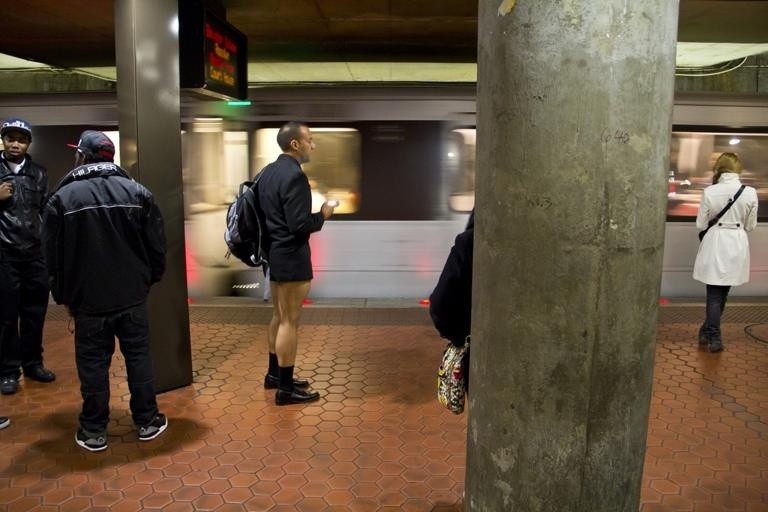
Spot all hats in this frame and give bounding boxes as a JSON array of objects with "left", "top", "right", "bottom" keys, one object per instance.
[
  {"left": 67, "top": 130, "right": 115, "bottom": 161},
  {"left": 0, "top": 117, "right": 33, "bottom": 140}
]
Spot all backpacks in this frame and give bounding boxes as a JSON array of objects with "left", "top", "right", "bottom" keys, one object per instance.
[{"left": 224, "top": 163, "right": 270, "bottom": 268}]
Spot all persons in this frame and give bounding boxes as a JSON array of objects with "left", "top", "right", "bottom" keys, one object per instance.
[
  {"left": 429, "top": 207, "right": 474, "bottom": 397},
  {"left": 253, "top": 120, "right": 340, "bottom": 405},
  {"left": 41, "top": 130, "right": 169, "bottom": 452},
  {"left": 692, "top": 152, "right": 758, "bottom": 352},
  {"left": 0, "top": 117, "right": 56, "bottom": 394}
]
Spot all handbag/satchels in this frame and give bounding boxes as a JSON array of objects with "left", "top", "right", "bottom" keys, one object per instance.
[{"left": 437, "top": 335, "right": 473, "bottom": 416}]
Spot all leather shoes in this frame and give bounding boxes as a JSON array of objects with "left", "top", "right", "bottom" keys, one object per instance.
[
  {"left": 275, "top": 387, "right": 319, "bottom": 405},
  {"left": 0, "top": 375, "right": 18, "bottom": 394},
  {"left": 25, "top": 368, "right": 56, "bottom": 382},
  {"left": 265, "top": 373, "right": 309, "bottom": 388}
]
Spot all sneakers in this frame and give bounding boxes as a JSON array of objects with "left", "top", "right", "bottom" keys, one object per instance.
[
  {"left": 698, "top": 334, "right": 722, "bottom": 353},
  {"left": 74, "top": 430, "right": 108, "bottom": 451},
  {"left": 138, "top": 413, "right": 168, "bottom": 441}
]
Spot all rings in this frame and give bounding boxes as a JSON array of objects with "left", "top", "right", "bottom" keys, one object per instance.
[{"left": 8, "top": 189, "right": 10, "bottom": 193}]
